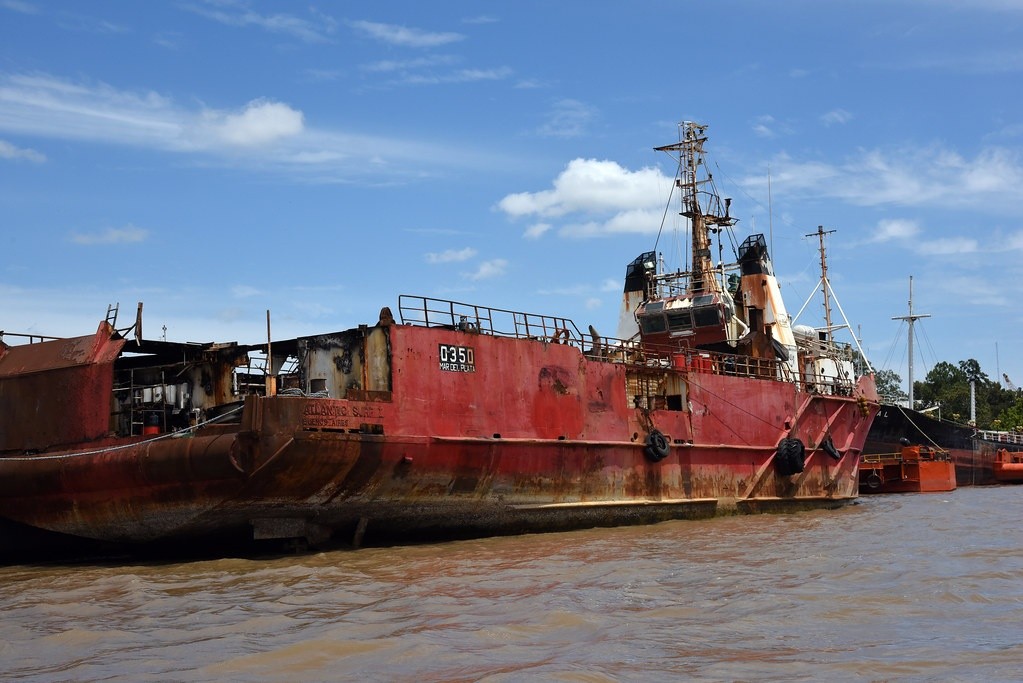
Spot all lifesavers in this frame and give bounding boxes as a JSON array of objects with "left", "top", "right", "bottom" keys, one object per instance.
[
  {"left": 824, "top": 441, "right": 840, "bottom": 460},
  {"left": 866, "top": 475, "right": 882, "bottom": 489},
  {"left": 553, "top": 329, "right": 570, "bottom": 345},
  {"left": 777, "top": 438, "right": 806, "bottom": 476},
  {"left": 645, "top": 432, "right": 670, "bottom": 463}
]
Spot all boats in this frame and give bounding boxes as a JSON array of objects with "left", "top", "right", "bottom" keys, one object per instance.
[
  {"left": 859, "top": 275, "right": 1023, "bottom": 493},
  {"left": 991, "top": 447, "right": 1023, "bottom": 483},
  {"left": 0, "top": 121, "right": 880, "bottom": 557}
]
